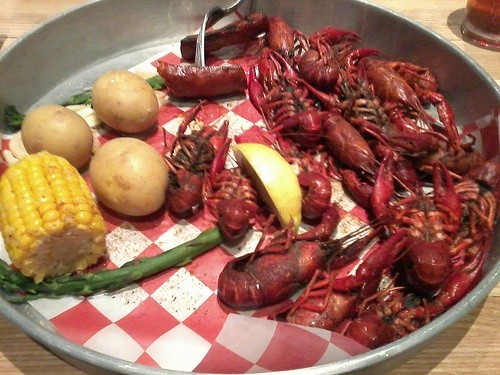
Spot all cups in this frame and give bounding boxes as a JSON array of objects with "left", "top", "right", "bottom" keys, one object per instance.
[{"left": 460, "top": 0, "right": 500, "bottom": 51}]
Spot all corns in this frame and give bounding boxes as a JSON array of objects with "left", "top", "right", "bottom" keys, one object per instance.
[{"left": 1, "top": 151, "right": 109, "bottom": 283}]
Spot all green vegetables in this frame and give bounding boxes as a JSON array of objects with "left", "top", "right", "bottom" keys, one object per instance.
[
  {"left": 0, "top": 227, "right": 225, "bottom": 304},
  {"left": 4, "top": 73, "right": 167, "bottom": 134}
]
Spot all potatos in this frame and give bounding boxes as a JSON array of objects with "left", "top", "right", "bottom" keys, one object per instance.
[
  {"left": 21, "top": 69, "right": 169, "bottom": 217},
  {"left": 231, "top": 143, "right": 303, "bottom": 235}
]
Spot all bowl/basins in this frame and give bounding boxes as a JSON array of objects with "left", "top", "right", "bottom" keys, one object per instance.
[{"left": 1, "top": 0, "right": 500, "bottom": 375}]
